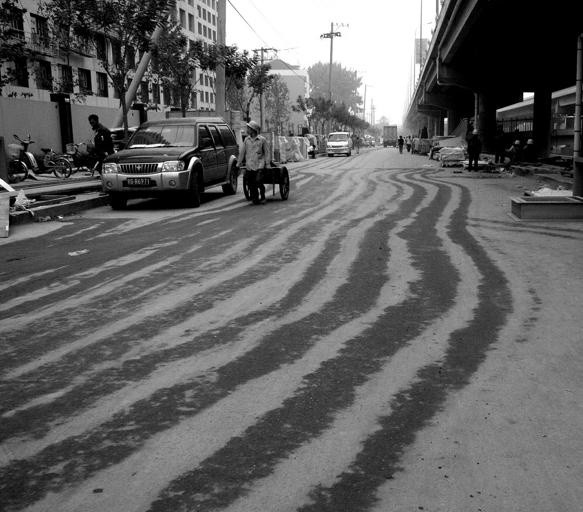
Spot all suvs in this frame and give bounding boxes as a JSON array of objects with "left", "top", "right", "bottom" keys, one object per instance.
[{"left": 101, "top": 115, "right": 241, "bottom": 210}]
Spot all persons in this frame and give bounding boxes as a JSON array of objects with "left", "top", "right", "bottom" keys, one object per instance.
[
  {"left": 398, "top": 135, "right": 420, "bottom": 154},
  {"left": 236, "top": 121, "right": 272, "bottom": 206},
  {"left": 493, "top": 125, "right": 539, "bottom": 163},
  {"left": 465, "top": 128, "right": 482, "bottom": 172},
  {"left": 88, "top": 114, "right": 115, "bottom": 175},
  {"left": 354, "top": 137, "right": 360, "bottom": 154}
]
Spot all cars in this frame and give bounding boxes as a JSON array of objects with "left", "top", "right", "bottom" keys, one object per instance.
[
  {"left": 365, "top": 136, "right": 375, "bottom": 148},
  {"left": 74, "top": 127, "right": 136, "bottom": 176}
]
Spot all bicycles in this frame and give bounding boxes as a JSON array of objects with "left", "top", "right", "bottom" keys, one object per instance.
[{"left": 59, "top": 143, "right": 101, "bottom": 175}]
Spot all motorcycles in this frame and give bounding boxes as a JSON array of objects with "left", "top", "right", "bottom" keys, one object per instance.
[{"left": 8, "top": 133, "right": 71, "bottom": 183}]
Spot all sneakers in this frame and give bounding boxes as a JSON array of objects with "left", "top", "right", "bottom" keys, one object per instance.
[{"left": 249, "top": 199, "right": 266, "bottom": 205}]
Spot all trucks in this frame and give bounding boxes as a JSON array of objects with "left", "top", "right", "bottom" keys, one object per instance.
[{"left": 383, "top": 126, "right": 396, "bottom": 148}]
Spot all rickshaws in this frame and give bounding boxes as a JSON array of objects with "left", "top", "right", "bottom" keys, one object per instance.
[{"left": 238, "top": 160, "right": 290, "bottom": 203}]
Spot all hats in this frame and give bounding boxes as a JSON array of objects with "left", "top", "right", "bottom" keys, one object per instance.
[{"left": 246, "top": 120, "right": 260, "bottom": 131}]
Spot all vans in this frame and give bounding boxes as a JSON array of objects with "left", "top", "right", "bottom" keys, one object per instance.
[{"left": 326, "top": 132, "right": 352, "bottom": 157}]
